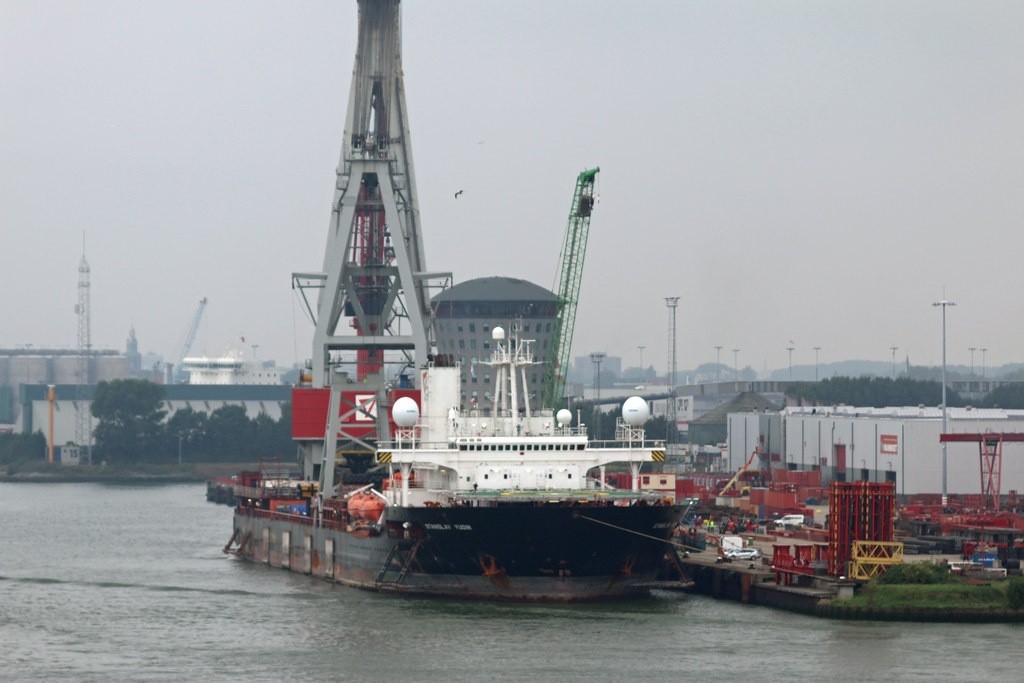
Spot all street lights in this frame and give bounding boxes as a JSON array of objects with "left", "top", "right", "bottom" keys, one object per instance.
[
  {"left": 179, "top": 437, "right": 184, "bottom": 464},
  {"left": 638, "top": 346, "right": 646, "bottom": 385},
  {"left": 932, "top": 284, "right": 956, "bottom": 505},
  {"left": 890, "top": 346, "right": 899, "bottom": 379},
  {"left": 25, "top": 344, "right": 32, "bottom": 384},
  {"left": 714, "top": 346, "right": 722, "bottom": 383},
  {"left": 732, "top": 349, "right": 740, "bottom": 381},
  {"left": 787, "top": 348, "right": 795, "bottom": 381},
  {"left": 813, "top": 347, "right": 822, "bottom": 381},
  {"left": 968, "top": 347, "right": 976, "bottom": 376},
  {"left": 980, "top": 349, "right": 988, "bottom": 377}
]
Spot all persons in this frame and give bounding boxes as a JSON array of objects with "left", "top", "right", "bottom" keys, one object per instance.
[
  {"left": 707, "top": 519, "right": 715, "bottom": 533},
  {"left": 393, "top": 469, "right": 414, "bottom": 488},
  {"left": 607, "top": 476, "right": 617, "bottom": 487}
]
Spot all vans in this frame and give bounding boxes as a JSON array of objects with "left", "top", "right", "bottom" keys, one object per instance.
[{"left": 774, "top": 514, "right": 804, "bottom": 527}]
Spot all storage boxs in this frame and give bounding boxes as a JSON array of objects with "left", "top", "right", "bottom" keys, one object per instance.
[{"left": 604, "top": 468, "right": 827, "bottom": 524}]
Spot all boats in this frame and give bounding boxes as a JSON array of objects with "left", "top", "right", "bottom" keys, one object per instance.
[{"left": 222, "top": 313, "right": 695, "bottom": 603}]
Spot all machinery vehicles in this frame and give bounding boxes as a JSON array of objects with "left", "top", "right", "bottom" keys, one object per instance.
[{"left": 712, "top": 446, "right": 763, "bottom": 497}]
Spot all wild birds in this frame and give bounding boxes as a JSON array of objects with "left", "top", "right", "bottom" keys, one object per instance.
[{"left": 454, "top": 190, "right": 463, "bottom": 199}]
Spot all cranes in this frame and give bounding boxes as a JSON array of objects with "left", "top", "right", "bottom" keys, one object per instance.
[
  {"left": 541, "top": 166, "right": 600, "bottom": 410},
  {"left": 173, "top": 297, "right": 207, "bottom": 384}
]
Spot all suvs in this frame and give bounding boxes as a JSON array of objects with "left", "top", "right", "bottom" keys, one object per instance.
[{"left": 724, "top": 548, "right": 760, "bottom": 561}]
